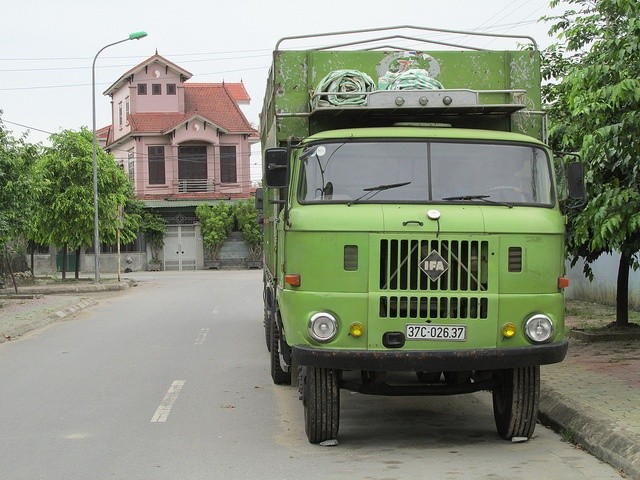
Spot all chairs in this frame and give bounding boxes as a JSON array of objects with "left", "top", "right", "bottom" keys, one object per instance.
[
  {"left": 448, "top": 175, "right": 486, "bottom": 202},
  {"left": 346, "top": 174, "right": 384, "bottom": 200}
]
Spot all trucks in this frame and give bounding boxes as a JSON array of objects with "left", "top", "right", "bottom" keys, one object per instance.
[{"left": 257, "top": 24, "right": 590, "bottom": 446}]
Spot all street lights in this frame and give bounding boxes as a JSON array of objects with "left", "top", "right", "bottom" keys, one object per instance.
[{"left": 83, "top": 30, "right": 150, "bottom": 286}]
